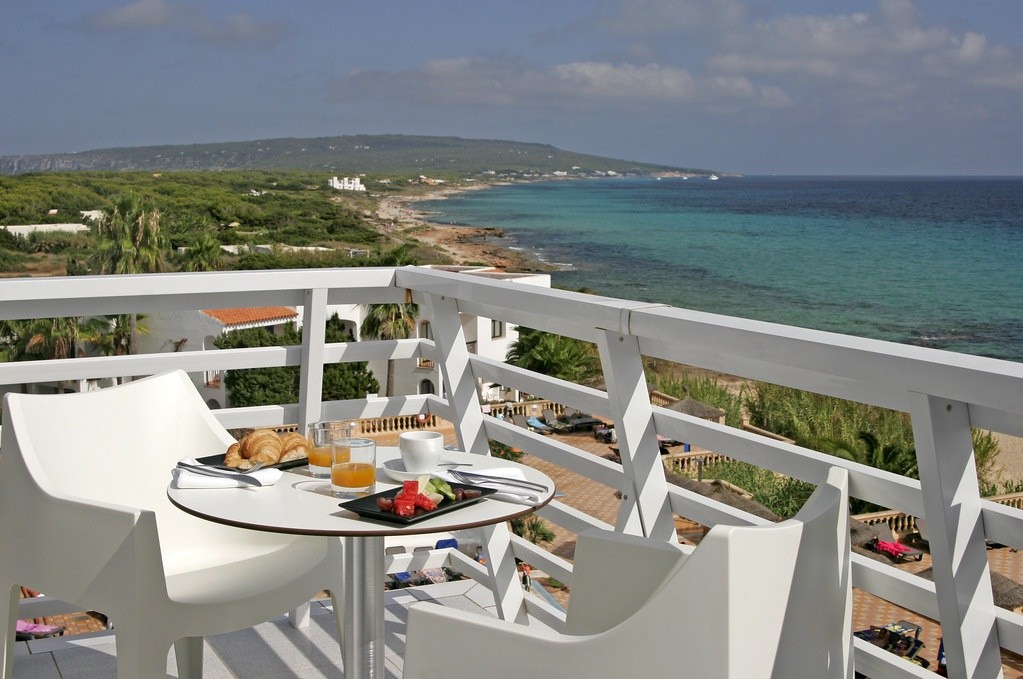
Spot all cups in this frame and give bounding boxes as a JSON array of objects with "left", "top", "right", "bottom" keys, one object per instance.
[
  {"left": 399, "top": 431, "right": 444, "bottom": 472},
  {"left": 330, "top": 437, "right": 376, "bottom": 500},
  {"left": 307, "top": 421, "right": 352, "bottom": 478}
]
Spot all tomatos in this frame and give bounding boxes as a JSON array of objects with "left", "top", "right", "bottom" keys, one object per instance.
[{"left": 393, "top": 480, "right": 436, "bottom": 517}]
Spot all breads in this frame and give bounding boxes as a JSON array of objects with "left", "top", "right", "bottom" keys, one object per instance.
[{"left": 222, "top": 427, "right": 310, "bottom": 471}]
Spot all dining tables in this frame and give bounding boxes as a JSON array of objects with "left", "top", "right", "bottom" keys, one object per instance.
[{"left": 165, "top": 444, "right": 557, "bottom": 679}]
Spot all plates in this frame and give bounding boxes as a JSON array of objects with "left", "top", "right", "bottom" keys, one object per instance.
[
  {"left": 338, "top": 481, "right": 499, "bottom": 525},
  {"left": 195, "top": 453, "right": 308, "bottom": 474},
  {"left": 382, "top": 458, "right": 458, "bottom": 482}
]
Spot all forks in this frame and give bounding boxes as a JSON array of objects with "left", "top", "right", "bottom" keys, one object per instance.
[
  {"left": 447, "top": 469, "right": 543, "bottom": 494},
  {"left": 195, "top": 461, "right": 265, "bottom": 474}
]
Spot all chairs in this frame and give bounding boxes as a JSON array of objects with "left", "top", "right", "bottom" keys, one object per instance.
[
  {"left": 866, "top": 522, "right": 924, "bottom": 562},
  {"left": 402, "top": 516, "right": 806, "bottom": 678},
  {"left": 914, "top": 518, "right": 929, "bottom": 546},
  {"left": 497, "top": 407, "right": 685, "bottom": 461},
  {"left": 562, "top": 464, "right": 857, "bottom": 679},
  {"left": 852, "top": 620, "right": 947, "bottom": 676},
  {"left": 0, "top": 368, "right": 354, "bottom": 679}
]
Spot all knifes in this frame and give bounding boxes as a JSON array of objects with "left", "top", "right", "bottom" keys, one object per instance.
[
  {"left": 177, "top": 461, "right": 262, "bottom": 487},
  {"left": 448, "top": 470, "right": 548, "bottom": 492}
]
